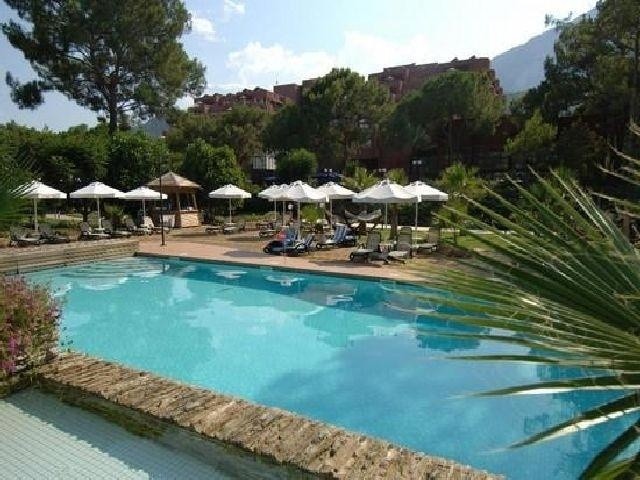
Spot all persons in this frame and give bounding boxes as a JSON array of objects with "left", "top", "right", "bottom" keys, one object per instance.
[{"left": 263, "top": 230, "right": 286, "bottom": 253}]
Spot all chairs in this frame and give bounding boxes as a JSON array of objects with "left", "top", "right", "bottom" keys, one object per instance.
[
  {"left": 9, "top": 216, "right": 170, "bottom": 248},
  {"left": 205, "top": 215, "right": 441, "bottom": 268}
]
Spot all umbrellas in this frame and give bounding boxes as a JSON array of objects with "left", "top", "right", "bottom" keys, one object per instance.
[
  {"left": 46, "top": 258, "right": 171, "bottom": 298},
  {"left": 7, "top": 178, "right": 68, "bottom": 235},
  {"left": 257, "top": 178, "right": 449, "bottom": 245},
  {"left": 214, "top": 267, "right": 248, "bottom": 280},
  {"left": 120, "top": 185, "right": 169, "bottom": 218},
  {"left": 384, "top": 295, "right": 435, "bottom": 317},
  {"left": 69, "top": 181, "right": 124, "bottom": 228},
  {"left": 208, "top": 183, "right": 252, "bottom": 223},
  {"left": 261, "top": 269, "right": 310, "bottom": 286}
]
